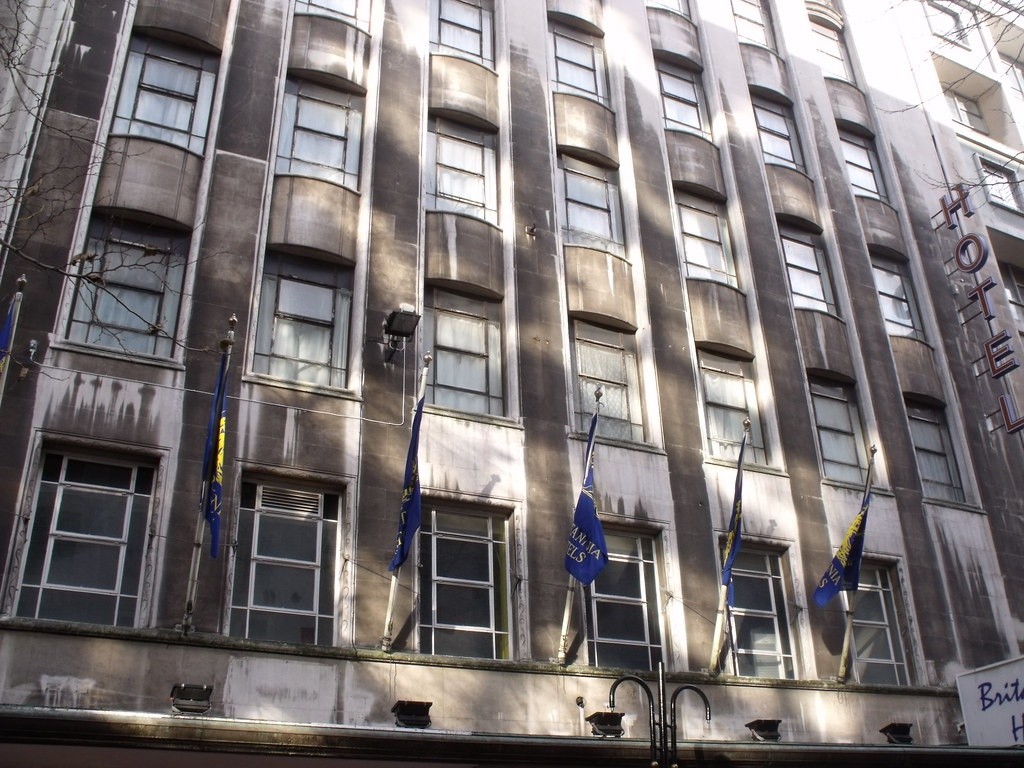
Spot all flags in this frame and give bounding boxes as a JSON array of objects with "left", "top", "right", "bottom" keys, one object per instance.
[
  {"left": 387, "top": 392, "right": 426, "bottom": 572},
  {"left": 721, "top": 434, "right": 745, "bottom": 607},
  {"left": 564, "top": 414, "right": 609, "bottom": 586},
  {"left": 201, "top": 350, "right": 228, "bottom": 561},
  {"left": 812, "top": 465, "right": 872, "bottom": 609}
]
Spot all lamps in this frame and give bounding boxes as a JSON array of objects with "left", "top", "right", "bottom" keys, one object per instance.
[
  {"left": 879, "top": 722, "right": 913, "bottom": 745},
  {"left": 390, "top": 700, "right": 433, "bottom": 728},
  {"left": 169, "top": 683, "right": 214, "bottom": 719},
  {"left": 745, "top": 719, "right": 782, "bottom": 742},
  {"left": 584, "top": 712, "right": 625, "bottom": 737},
  {"left": 381, "top": 303, "right": 422, "bottom": 363}
]
